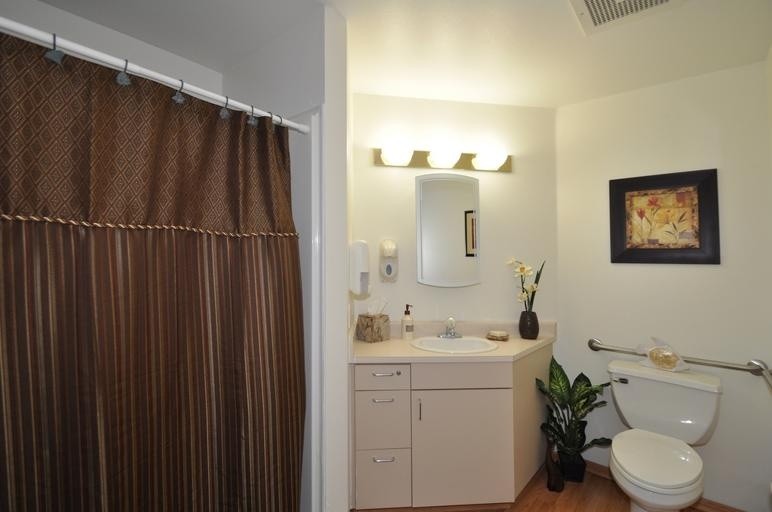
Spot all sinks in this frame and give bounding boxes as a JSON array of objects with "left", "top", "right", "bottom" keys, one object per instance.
[{"left": 410, "top": 336, "right": 497, "bottom": 354}]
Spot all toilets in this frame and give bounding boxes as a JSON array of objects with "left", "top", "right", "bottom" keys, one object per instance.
[{"left": 606, "top": 361, "right": 722, "bottom": 512}]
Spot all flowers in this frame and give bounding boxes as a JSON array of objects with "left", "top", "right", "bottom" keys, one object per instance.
[{"left": 506, "top": 257, "right": 546, "bottom": 312}]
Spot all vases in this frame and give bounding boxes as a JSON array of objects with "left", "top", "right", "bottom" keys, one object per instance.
[{"left": 519, "top": 310, "right": 539, "bottom": 340}]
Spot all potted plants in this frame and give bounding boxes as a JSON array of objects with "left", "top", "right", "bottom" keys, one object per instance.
[{"left": 536, "top": 355, "right": 613, "bottom": 483}]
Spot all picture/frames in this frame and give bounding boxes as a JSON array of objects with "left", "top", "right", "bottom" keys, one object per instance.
[
  {"left": 608, "top": 169, "right": 721, "bottom": 266},
  {"left": 464, "top": 210, "right": 475, "bottom": 256}
]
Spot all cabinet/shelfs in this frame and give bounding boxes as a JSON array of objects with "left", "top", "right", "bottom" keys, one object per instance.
[{"left": 349, "top": 344, "right": 553, "bottom": 509}]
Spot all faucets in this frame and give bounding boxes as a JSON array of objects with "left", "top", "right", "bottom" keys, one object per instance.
[{"left": 445, "top": 317, "right": 456, "bottom": 337}]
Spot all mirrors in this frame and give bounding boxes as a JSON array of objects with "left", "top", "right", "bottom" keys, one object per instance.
[{"left": 416, "top": 172, "right": 482, "bottom": 288}]
